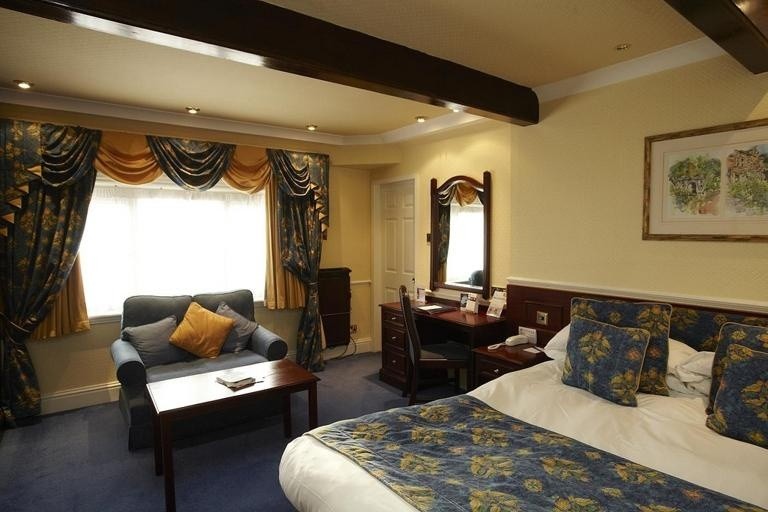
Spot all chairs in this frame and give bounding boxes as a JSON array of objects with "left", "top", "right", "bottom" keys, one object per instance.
[{"left": 397, "top": 282, "right": 472, "bottom": 409}]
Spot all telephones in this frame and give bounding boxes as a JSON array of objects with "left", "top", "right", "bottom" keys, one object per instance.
[{"left": 505, "top": 335, "right": 529, "bottom": 347}]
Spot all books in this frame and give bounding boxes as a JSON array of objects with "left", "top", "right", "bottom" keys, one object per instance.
[
  {"left": 413, "top": 302, "right": 458, "bottom": 318},
  {"left": 216, "top": 370, "right": 257, "bottom": 389}
]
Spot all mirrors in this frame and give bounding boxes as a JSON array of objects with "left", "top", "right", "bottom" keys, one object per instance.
[{"left": 427, "top": 170, "right": 494, "bottom": 300}]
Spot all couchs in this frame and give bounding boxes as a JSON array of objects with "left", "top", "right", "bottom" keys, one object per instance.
[{"left": 108, "top": 288, "right": 290, "bottom": 453}]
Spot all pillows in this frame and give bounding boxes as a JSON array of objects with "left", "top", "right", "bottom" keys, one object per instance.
[
  {"left": 122, "top": 315, "right": 188, "bottom": 369},
  {"left": 214, "top": 300, "right": 258, "bottom": 357},
  {"left": 541, "top": 294, "right": 768, "bottom": 451},
  {"left": 164, "top": 300, "right": 236, "bottom": 363}
]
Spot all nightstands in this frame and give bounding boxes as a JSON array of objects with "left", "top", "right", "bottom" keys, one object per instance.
[{"left": 470, "top": 334, "right": 554, "bottom": 389}]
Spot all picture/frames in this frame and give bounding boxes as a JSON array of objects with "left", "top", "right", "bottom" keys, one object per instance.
[{"left": 640, "top": 116, "right": 768, "bottom": 243}]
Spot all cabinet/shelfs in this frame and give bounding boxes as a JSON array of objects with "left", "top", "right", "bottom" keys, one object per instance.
[{"left": 316, "top": 267, "right": 352, "bottom": 349}]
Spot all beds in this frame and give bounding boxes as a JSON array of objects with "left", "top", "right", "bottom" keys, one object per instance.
[{"left": 278, "top": 294, "right": 768, "bottom": 512}]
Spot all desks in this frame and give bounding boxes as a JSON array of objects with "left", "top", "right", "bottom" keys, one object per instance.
[
  {"left": 145, "top": 356, "right": 322, "bottom": 511},
  {"left": 378, "top": 297, "right": 511, "bottom": 396}
]
